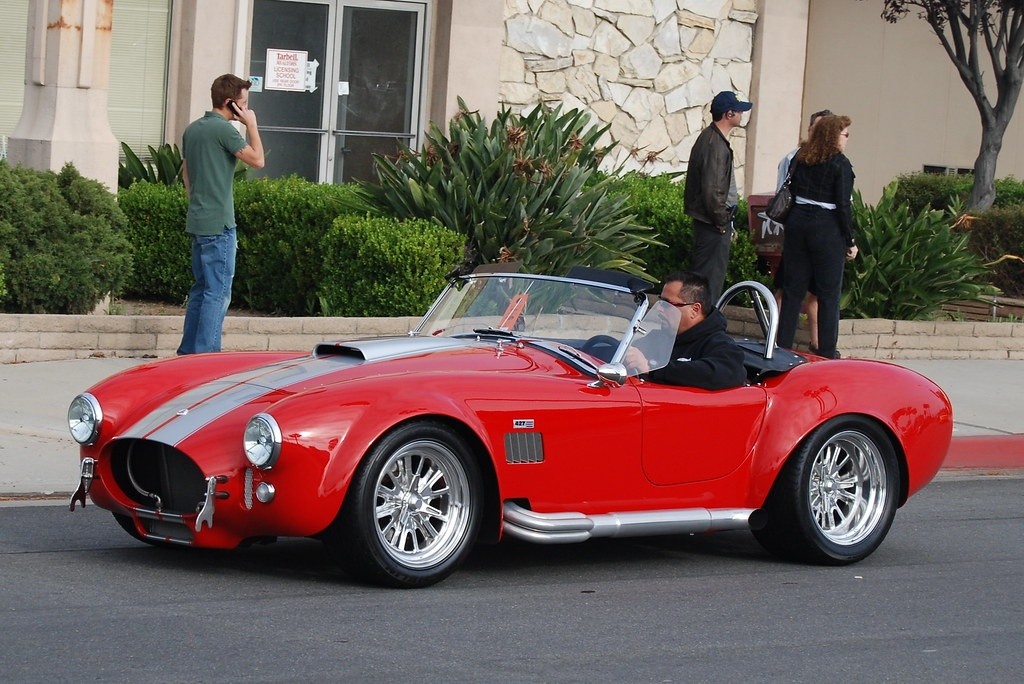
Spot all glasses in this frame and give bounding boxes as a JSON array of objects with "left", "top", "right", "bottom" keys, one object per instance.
[
  {"left": 658, "top": 295, "right": 696, "bottom": 308},
  {"left": 839, "top": 132, "right": 849, "bottom": 138}
]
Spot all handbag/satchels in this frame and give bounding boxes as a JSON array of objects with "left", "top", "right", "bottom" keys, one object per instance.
[{"left": 766, "top": 148, "right": 803, "bottom": 224}]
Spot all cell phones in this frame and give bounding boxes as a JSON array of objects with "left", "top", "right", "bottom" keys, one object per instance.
[{"left": 227, "top": 100, "right": 242, "bottom": 116}]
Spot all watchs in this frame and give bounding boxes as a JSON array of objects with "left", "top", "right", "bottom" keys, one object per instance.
[{"left": 647, "top": 358, "right": 659, "bottom": 377}]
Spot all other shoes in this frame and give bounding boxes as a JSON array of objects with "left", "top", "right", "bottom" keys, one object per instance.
[
  {"left": 826, "top": 351, "right": 841, "bottom": 360},
  {"left": 807, "top": 342, "right": 819, "bottom": 355}
]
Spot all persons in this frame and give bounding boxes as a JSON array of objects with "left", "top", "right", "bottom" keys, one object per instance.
[
  {"left": 775, "top": 109, "right": 859, "bottom": 359},
  {"left": 684, "top": 92, "right": 753, "bottom": 306},
  {"left": 624, "top": 272, "right": 748, "bottom": 392},
  {"left": 178, "top": 73, "right": 265, "bottom": 353}
]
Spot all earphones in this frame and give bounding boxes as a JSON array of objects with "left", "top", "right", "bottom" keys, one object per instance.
[{"left": 728, "top": 114, "right": 735, "bottom": 117}]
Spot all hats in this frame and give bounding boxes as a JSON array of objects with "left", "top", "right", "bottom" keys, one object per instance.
[{"left": 711, "top": 91, "right": 752, "bottom": 114}]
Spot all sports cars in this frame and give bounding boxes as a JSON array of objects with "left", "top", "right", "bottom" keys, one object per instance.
[{"left": 65, "top": 264, "right": 955, "bottom": 588}]
